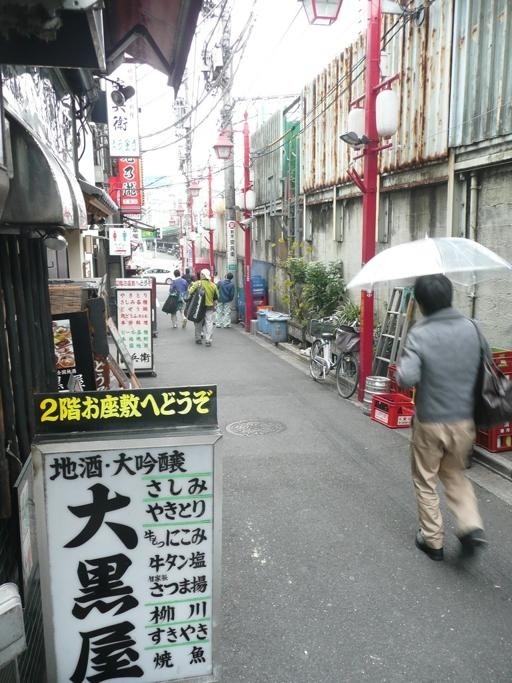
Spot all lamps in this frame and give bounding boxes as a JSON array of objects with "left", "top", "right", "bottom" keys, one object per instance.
[
  {"left": 93, "top": 71, "right": 136, "bottom": 106},
  {"left": 34, "top": 228, "right": 68, "bottom": 250}
]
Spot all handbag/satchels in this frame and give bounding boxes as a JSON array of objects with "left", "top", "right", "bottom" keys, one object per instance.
[
  {"left": 161, "top": 290, "right": 181, "bottom": 315},
  {"left": 468, "top": 319, "right": 512, "bottom": 431},
  {"left": 184, "top": 287, "right": 207, "bottom": 322}
]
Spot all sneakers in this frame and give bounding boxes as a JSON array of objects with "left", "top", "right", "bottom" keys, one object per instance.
[
  {"left": 171, "top": 319, "right": 187, "bottom": 329},
  {"left": 196, "top": 335, "right": 211, "bottom": 347}
]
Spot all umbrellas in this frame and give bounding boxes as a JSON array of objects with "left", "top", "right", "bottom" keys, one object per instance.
[{"left": 344, "top": 232, "right": 511, "bottom": 291}]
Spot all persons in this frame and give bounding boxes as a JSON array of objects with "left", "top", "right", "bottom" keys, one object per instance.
[
  {"left": 215, "top": 272, "right": 236, "bottom": 329},
  {"left": 188, "top": 268, "right": 220, "bottom": 345},
  {"left": 392, "top": 275, "right": 492, "bottom": 563},
  {"left": 182, "top": 267, "right": 222, "bottom": 285},
  {"left": 169, "top": 269, "right": 187, "bottom": 329}
]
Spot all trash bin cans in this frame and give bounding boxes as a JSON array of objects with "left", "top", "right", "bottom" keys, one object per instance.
[{"left": 256, "top": 310, "right": 291, "bottom": 343}]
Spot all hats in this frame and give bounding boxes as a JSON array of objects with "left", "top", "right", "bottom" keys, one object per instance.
[{"left": 200, "top": 269, "right": 212, "bottom": 280}]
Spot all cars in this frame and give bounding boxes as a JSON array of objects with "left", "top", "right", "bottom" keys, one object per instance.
[{"left": 142, "top": 267, "right": 176, "bottom": 285}]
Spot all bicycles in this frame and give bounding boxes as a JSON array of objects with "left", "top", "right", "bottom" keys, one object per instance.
[{"left": 309, "top": 313, "right": 360, "bottom": 399}]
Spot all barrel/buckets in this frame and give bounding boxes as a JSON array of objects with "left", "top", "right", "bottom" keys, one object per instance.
[{"left": 362, "top": 375, "right": 392, "bottom": 415}]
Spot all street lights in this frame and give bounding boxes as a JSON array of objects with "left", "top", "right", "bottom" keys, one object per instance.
[
  {"left": 212, "top": 110, "right": 256, "bottom": 332},
  {"left": 169, "top": 165, "right": 216, "bottom": 280},
  {"left": 300, "top": 0, "right": 400, "bottom": 403}
]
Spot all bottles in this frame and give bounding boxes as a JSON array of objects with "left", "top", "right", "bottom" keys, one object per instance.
[
  {"left": 376, "top": 402, "right": 388, "bottom": 411},
  {"left": 506, "top": 436, "right": 512, "bottom": 448},
  {"left": 496, "top": 437, "right": 502, "bottom": 448}
]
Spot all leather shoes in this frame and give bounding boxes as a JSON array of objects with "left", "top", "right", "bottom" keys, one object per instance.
[
  {"left": 459, "top": 528, "right": 488, "bottom": 550},
  {"left": 415, "top": 530, "right": 444, "bottom": 561}
]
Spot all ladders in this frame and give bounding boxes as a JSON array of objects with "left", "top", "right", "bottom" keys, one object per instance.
[{"left": 372, "top": 286, "right": 415, "bottom": 379}]
